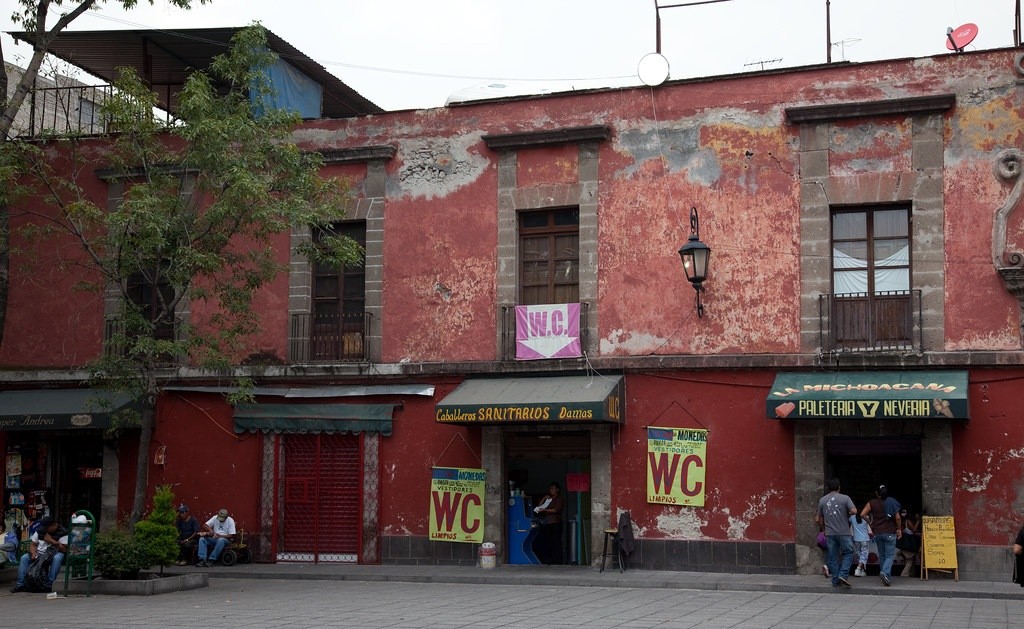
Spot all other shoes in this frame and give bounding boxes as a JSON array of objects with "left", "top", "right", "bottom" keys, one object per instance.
[
  {"left": 45, "top": 583, "right": 52, "bottom": 592},
  {"left": 833, "top": 576, "right": 851, "bottom": 587},
  {"left": 10, "top": 585, "right": 26, "bottom": 593}
]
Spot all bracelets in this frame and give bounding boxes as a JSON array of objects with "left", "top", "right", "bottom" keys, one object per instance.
[{"left": 186, "top": 539, "right": 188, "bottom": 542}]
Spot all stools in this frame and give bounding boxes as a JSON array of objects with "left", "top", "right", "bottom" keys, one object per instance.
[{"left": 39, "top": 557, "right": 75, "bottom": 577}]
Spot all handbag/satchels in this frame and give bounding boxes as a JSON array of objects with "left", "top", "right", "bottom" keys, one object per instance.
[
  {"left": 895, "top": 531, "right": 921, "bottom": 561},
  {"left": 24, "top": 543, "right": 60, "bottom": 593}
]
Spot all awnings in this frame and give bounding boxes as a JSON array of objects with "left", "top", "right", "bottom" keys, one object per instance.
[
  {"left": 231, "top": 403, "right": 402, "bottom": 437},
  {"left": 435, "top": 374, "right": 625, "bottom": 427},
  {"left": 766, "top": 369, "right": 970, "bottom": 419},
  {"left": 0, "top": 388, "right": 154, "bottom": 431}
]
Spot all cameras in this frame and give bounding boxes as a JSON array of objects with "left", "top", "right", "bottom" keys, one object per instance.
[{"left": 37, "top": 527, "right": 48, "bottom": 540}]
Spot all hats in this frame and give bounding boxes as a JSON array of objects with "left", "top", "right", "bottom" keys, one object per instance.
[
  {"left": 177, "top": 506, "right": 187, "bottom": 512},
  {"left": 218, "top": 509, "right": 228, "bottom": 521}
]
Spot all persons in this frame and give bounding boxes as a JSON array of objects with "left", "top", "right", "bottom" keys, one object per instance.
[
  {"left": 10, "top": 517, "right": 68, "bottom": 593},
  {"left": 174, "top": 505, "right": 200, "bottom": 565},
  {"left": 1013, "top": 525, "right": 1024, "bottom": 555},
  {"left": 0, "top": 521, "right": 19, "bottom": 565},
  {"left": 816, "top": 476, "right": 921, "bottom": 588},
  {"left": 195, "top": 509, "right": 236, "bottom": 567},
  {"left": 539, "top": 482, "right": 565, "bottom": 565}
]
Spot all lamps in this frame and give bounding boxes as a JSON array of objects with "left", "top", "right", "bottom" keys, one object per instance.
[{"left": 677, "top": 207, "right": 712, "bottom": 319}]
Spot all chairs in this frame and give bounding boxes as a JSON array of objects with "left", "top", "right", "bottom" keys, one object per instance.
[
  {"left": 2, "top": 540, "right": 27, "bottom": 567},
  {"left": 599, "top": 511, "right": 630, "bottom": 574}
]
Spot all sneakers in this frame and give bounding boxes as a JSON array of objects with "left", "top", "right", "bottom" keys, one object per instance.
[
  {"left": 822, "top": 565, "right": 830, "bottom": 578},
  {"left": 195, "top": 559, "right": 216, "bottom": 567},
  {"left": 879, "top": 573, "right": 890, "bottom": 586},
  {"left": 854, "top": 569, "right": 866, "bottom": 577},
  {"left": 174, "top": 560, "right": 187, "bottom": 566}
]
